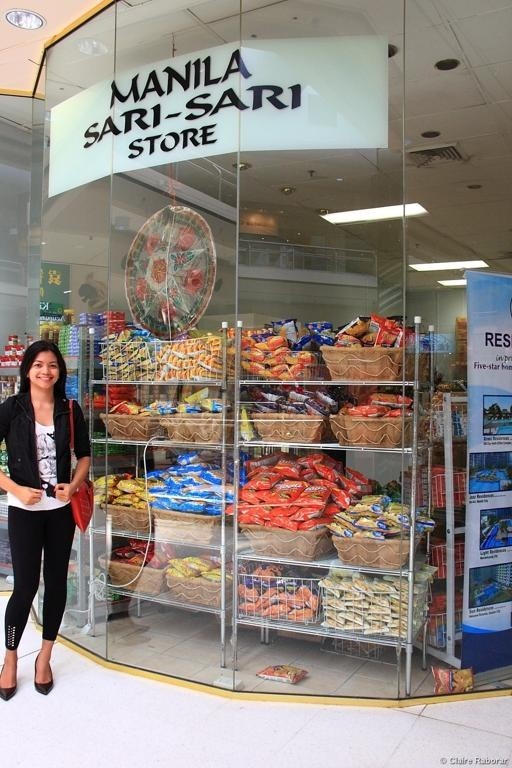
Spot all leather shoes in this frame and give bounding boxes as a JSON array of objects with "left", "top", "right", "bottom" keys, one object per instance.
[
  {"left": 0, "top": 665, "right": 17, "bottom": 701},
  {"left": 34, "top": 654, "right": 53, "bottom": 695}
]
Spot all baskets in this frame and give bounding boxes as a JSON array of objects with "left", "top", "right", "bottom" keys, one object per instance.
[
  {"left": 100, "top": 401, "right": 467, "bottom": 448},
  {"left": 97, "top": 547, "right": 463, "bottom": 648},
  {"left": 98, "top": 336, "right": 426, "bottom": 381},
  {"left": 100, "top": 466, "right": 466, "bottom": 578}
]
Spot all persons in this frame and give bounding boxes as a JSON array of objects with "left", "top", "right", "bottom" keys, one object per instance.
[{"left": 0, "top": 340, "right": 91, "bottom": 701}]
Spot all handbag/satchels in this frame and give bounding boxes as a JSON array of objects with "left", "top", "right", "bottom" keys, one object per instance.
[{"left": 70, "top": 479, "right": 93, "bottom": 533}]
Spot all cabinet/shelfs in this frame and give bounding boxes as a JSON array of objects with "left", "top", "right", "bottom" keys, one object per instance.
[
  {"left": 232, "top": 314, "right": 437, "bottom": 697},
  {"left": 88, "top": 321, "right": 231, "bottom": 670},
  {"left": 407, "top": 385, "right": 470, "bottom": 670},
  {"left": 38, "top": 317, "right": 152, "bottom": 627}
]
